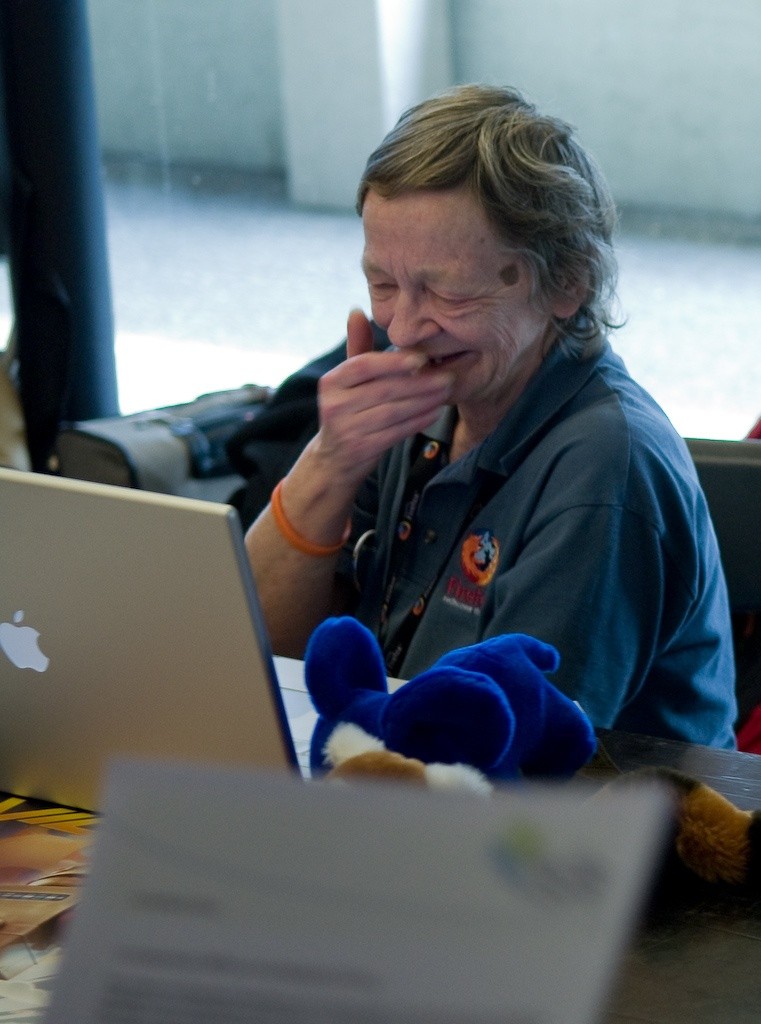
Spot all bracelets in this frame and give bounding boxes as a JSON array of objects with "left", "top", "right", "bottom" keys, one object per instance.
[{"left": 271, "top": 479, "right": 351, "bottom": 556}]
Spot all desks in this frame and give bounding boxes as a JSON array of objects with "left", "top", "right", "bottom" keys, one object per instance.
[{"left": 0, "top": 654, "right": 761, "bottom": 1024}]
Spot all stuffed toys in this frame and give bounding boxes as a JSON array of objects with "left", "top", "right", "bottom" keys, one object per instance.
[{"left": 304, "top": 615, "right": 761, "bottom": 906}]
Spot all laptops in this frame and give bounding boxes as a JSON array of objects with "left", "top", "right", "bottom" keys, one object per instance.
[{"left": 0, "top": 466, "right": 411, "bottom": 813}]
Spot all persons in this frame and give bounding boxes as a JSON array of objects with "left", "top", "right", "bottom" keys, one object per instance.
[{"left": 241, "top": 84, "right": 738, "bottom": 753}]
